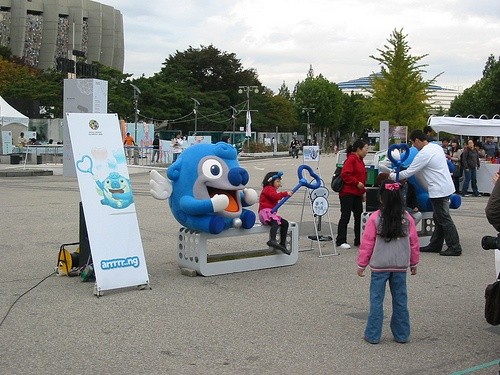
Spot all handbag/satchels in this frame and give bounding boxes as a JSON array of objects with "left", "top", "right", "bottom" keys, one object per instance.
[
  {"left": 453, "top": 156, "right": 463, "bottom": 178},
  {"left": 484, "top": 281, "right": 500, "bottom": 326}
]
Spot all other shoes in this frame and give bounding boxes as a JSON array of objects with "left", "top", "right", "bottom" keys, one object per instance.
[
  {"left": 266, "top": 239, "right": 282, "bottom": 249},
  {"left": 462, "top": 195, "right": 469, "bottom": 197},
  {"left": 473, "top": 194, "right": 481, "bottom": 197},
  {"left": 363, "top": 335, "right": 377, "bottom": 344},
  {"left": 278, "top": 243, "right": 290, "bottom": 255},
  {"left": 420, "top": 243, "right": 441, "bottom": 253},
  {"left": 440, "top": 248, "right": 461, "bottom": 256}
]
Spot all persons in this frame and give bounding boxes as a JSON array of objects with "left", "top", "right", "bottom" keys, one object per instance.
[
  {"left": 124, "top": 132, "right": 134, "bottom": 156},
  {"left": 336, "top": 140, "right": 369, "bottom": 249},
  {"left": 259, "top": 172, "right": 293, "bottom": 255},
  {"left": 28, "top": 138, "right": 36, "bottom": 145},
  {"left": 485, "top": 176, "right": 500, "bottom": 233},
  {"left": 151, "top": 136, "right": 160, "bottom": 162},
  {"left": 17, "top": 132, "right": 27, "bottom": 152},
  {"left": 48, "top": 139, "right": 53, "bottom": 144},
  {"left": 289, "top": 137, "right": 316, "bottom": 159},
  {"left": 442, "top": 135, "right": 500, "bottom": 197},
  {"left": 357, "top": 180, "right": 419, "bottom": 344},
  {"left": 423, "top": 126, "right": 437, "bottom": 143},
  {"left": 170, "top": 132, "right": 187, "bottom": 163},
  {"left": 387, "top": 130, "right": 462, "bottom": 256}
]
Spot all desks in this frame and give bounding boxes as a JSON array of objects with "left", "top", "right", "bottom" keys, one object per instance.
[{"left": 464, "top": 163, "right": 500, "bottom": 196}]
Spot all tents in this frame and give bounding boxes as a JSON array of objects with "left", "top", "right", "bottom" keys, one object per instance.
[{"left": 0, "top": 95, "right": 30, "bottom": 143}]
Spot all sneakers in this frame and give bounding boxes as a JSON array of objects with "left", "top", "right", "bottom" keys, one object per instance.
[{"left": 337, "top": 243, "right": 351, "bottom": 249}]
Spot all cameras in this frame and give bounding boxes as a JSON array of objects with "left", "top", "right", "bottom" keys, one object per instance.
[{"left": 482, "top": 232, "right": 500, "bottom": 250}]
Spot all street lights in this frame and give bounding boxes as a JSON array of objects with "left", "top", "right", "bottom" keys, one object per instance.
[
  {"left": 130, "top": 84, "right": 141, "bottom": 165},
  {"left": 191, "top": 98, "right": 200, "bottom": 136},
  {"left": 238, "top": 86, "right": 259, "bottom": 154},
  {"left": 301, "top": 108, "right": 316, "bottom": 146}
]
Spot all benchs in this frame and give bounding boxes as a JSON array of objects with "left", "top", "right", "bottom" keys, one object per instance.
[
  {"left": 360, "top": 213, "right": 436, "bottom": 245},
  {"left": 176, "top": 217, "right": 300, "bottom": 276}
]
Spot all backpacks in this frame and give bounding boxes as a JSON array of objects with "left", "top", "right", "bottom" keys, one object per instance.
[{"left": 331, "top": 167, "right": 343, "bottom": 192}]
